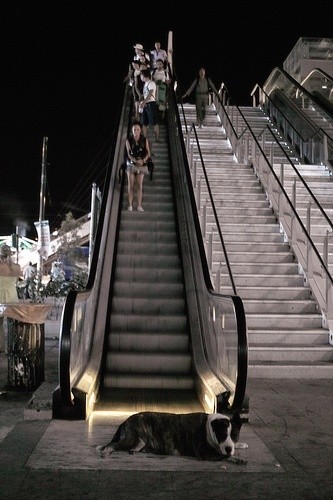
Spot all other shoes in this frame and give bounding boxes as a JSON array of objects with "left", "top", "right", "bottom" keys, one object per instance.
[
  {"left": 127, "top": 206, "right": 132, "bottom": 212},
  {"left": 198, "top": 123, "right": 203, "bottom": 129},
  {"left": 136, "top": 207, "right": 144, "bottom": 211},
  {"left": 156, "top": 139, "right": 162, "bottom": 144}
]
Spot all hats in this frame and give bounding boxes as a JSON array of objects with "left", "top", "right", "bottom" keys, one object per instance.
[{"left": 133, "top": 44, "right": 144, "bottom": 50}]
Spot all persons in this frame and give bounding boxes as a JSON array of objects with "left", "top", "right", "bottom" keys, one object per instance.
[
  {"left": 23, "top": 261, "right": 36, "bottom": 294},
  {"left": 183, "top": 67, "right": 223, "bottom": 129},
  {"left": 125, "top": 122, "right": 151, "bottom": 212},
  {"left": 122, "top": 42, "right": 170, "bottom": 143}
]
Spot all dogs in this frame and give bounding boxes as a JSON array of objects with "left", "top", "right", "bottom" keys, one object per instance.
[{"left": 95, "top": 411, "right": 248, "bottom": 466}]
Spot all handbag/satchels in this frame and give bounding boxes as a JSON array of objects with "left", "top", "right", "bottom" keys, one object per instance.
[{"left": 132, "top": 162, "right": 148, "bottom": 172}]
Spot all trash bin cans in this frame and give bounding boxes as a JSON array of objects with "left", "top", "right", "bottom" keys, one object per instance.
[{"left": 4, "top": 304, "right": 53, "bottom": 391}]
